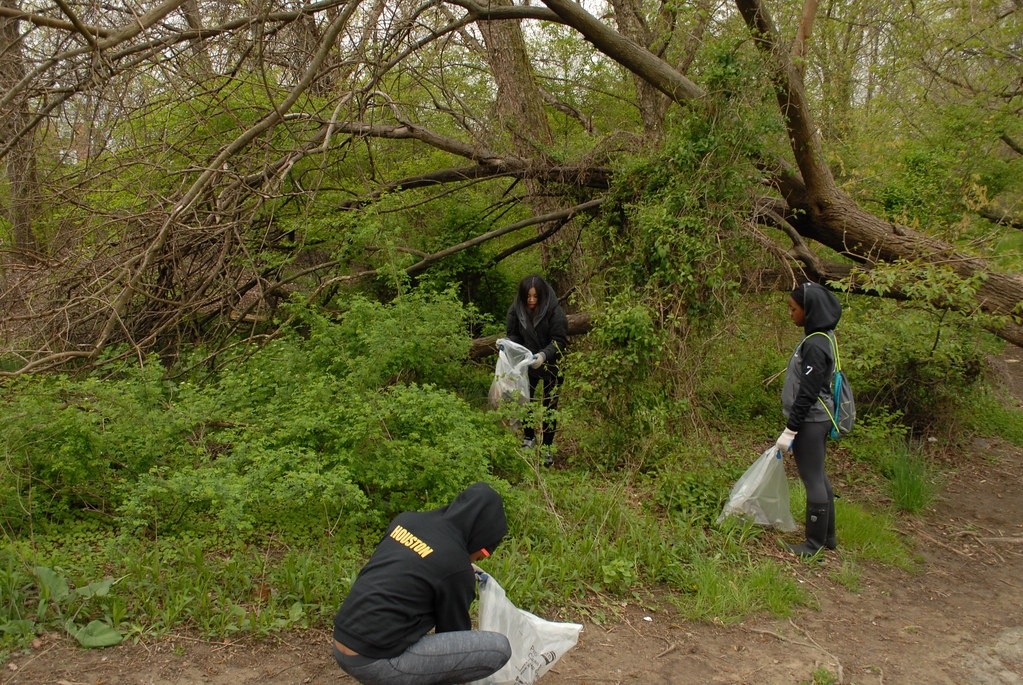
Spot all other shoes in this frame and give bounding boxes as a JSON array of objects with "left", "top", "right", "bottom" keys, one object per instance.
[
  {"left": 522, "top": 436, "right": 536, "bottom": 450},
  {"left": 544, "top": 453, "right": 554, "bottom": 468}
]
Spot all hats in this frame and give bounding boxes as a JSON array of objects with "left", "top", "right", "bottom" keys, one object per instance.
[
  {"left": 790, "top": 284, "right": 804, "bottom": 310},
  {"left": 480, "top": 538, "right": 502, "bottom": 557}
]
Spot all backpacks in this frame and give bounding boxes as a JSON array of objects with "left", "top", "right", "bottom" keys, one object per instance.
[{"left": 798, "top": 329, "right": 856, "bottom": 440}]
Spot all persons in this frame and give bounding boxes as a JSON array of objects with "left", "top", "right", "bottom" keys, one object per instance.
[
  {"left": 334, "top": 482, "right": 511, "bottom": 684},
  {"left": 774, "top": 282, "right": 843, "bottom": 561},
  {"left": 495, "top": 273, "right": 568, "bottom": 469}
]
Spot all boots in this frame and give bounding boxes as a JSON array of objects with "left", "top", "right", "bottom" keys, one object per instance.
[
  {"left": 826, "top": 508, "right": 837, "bottom": 549},
  {"left": 787, "top": 500, "right": 828, "bottom": 556}
]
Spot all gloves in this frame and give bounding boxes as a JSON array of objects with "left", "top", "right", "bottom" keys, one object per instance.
[
  {"left": 530, "top": 352, "right": 546, "bottom": 369},
  {"left": 471, "top": 563, "right": 490, "bottom": 590},
  {"left": 775, "top": 427, "right": 798, "bottom": 454},
  {"left": 495, "top": 338, "right": 508, "bottom": 352}
]
